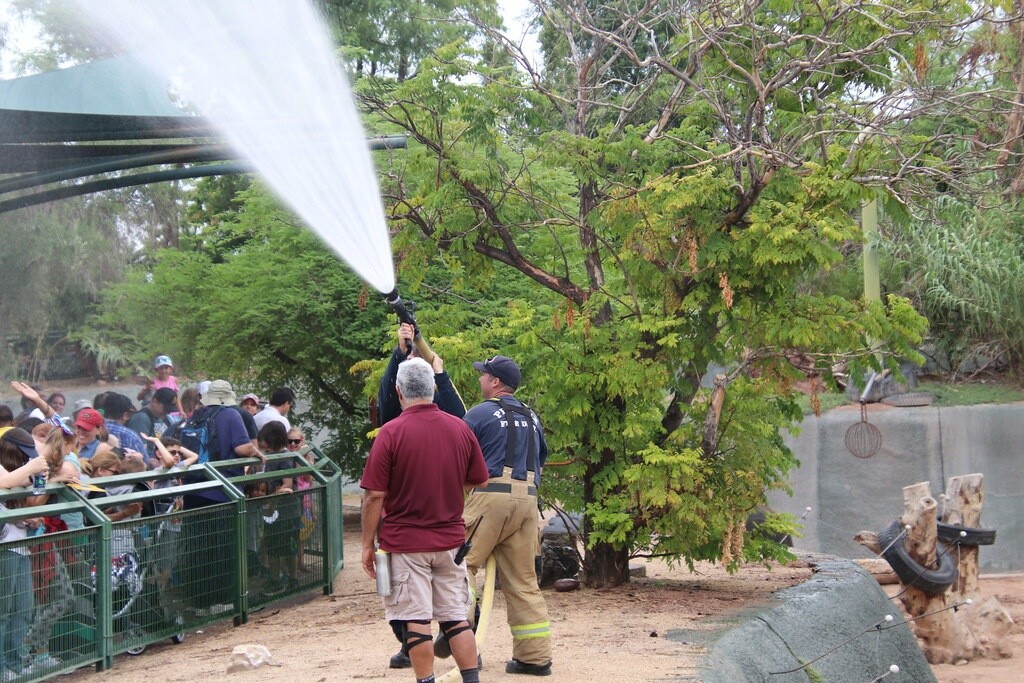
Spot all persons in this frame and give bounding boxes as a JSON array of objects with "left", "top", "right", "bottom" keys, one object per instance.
[
  {"left": 434, "top": 354, "right": 552, "bottom": 676},
  {"left": 359, "top": 357, "right": 490, "bottom": 683},
  {"left": 0, "top": 379, "right": 314, "bottom": 683},
  {"left": 378, "top": 322, "right": 469, "bottom": 669},
  {"left": 137, "top": 355, "right": 188, "bottom": 420}
]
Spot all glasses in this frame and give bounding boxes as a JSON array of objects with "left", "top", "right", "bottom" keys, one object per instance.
[
  {"left": 101, "top": 467, "right": 118, "bottom": 475},
  {"left": 485, "top": 358, "right": 502, "bottom": 381},
  {"left": 288, "top": 439, "right": 301, "bottom": 444},
  {"left": 169, "top": 450, "right": 183, "bottom": 456},
  {"left": 158, "top": 365, "right": 170, "bottom": 369}
]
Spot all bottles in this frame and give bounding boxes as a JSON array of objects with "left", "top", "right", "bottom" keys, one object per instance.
[
  {"left": 32, "top": 471, "right": 47, "bottom": 495},
  {"left": 374, "top": 548, "right": 391, "bottom": 597}
]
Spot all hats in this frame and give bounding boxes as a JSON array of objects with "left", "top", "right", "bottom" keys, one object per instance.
[
  {"left": 197, "top": 381, "right": 211, "bottom": 396},
  {"left": 155, "top": 387, "right": 179, "bottom": 412},
  {"left": 73, "top": 409, "right": 103, "bottom": 430},
  {"left": 74, "top": 399, "right": 92, "bottom": 412},
  {"left": 243, "top": 393, "right": 259, "bottom": 404},
  {"left": 200, "top": 380, "right": 237, "bottom": 407},
  {"left": 153, "top": 355, "right": 173, "bottom": 368},
  {"left": 4, "top": 435, "right": 39, "bottom": 458},
  {"left": 28, "top": 408, "right": 45, "bottom": 421},
  {"left": 473, "top": 355, "right": 520, "bottom": 389}
]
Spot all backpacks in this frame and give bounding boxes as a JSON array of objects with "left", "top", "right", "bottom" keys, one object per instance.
[{"left": 173, "top": 407, "right": 224, "bottom": 463}]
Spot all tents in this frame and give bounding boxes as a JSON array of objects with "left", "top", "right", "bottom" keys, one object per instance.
[{"left": 0, "top": 47, "right": 255, "bottom": 209}]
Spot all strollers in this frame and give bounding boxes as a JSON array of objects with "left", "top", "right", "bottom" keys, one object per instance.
[{"left": 42, "top": 481, "right": 188, "bottom": 668}]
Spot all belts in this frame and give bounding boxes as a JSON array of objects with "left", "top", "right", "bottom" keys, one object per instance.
[{"left": 474, "top": 483, "right": 538, "bottom": 496}]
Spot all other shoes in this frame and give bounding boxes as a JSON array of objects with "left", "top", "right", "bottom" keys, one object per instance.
[
  {"left": 390, "top": 645, "right": 411, "bottom": 666},
  {"left": 433, "top": 628, "right": 452, "bottom": 658},
  {"left": 262, "top": 578, "right": 298, "bottom": 596},
  {"left": 35, "top": 657, "right": 74, "bottom": 674},
  {"left": 506, "top": 659, "right": 552, "bottom": 676},
  {"left": 1, "top": 668, "right": 33, "bottom": 682}
]
